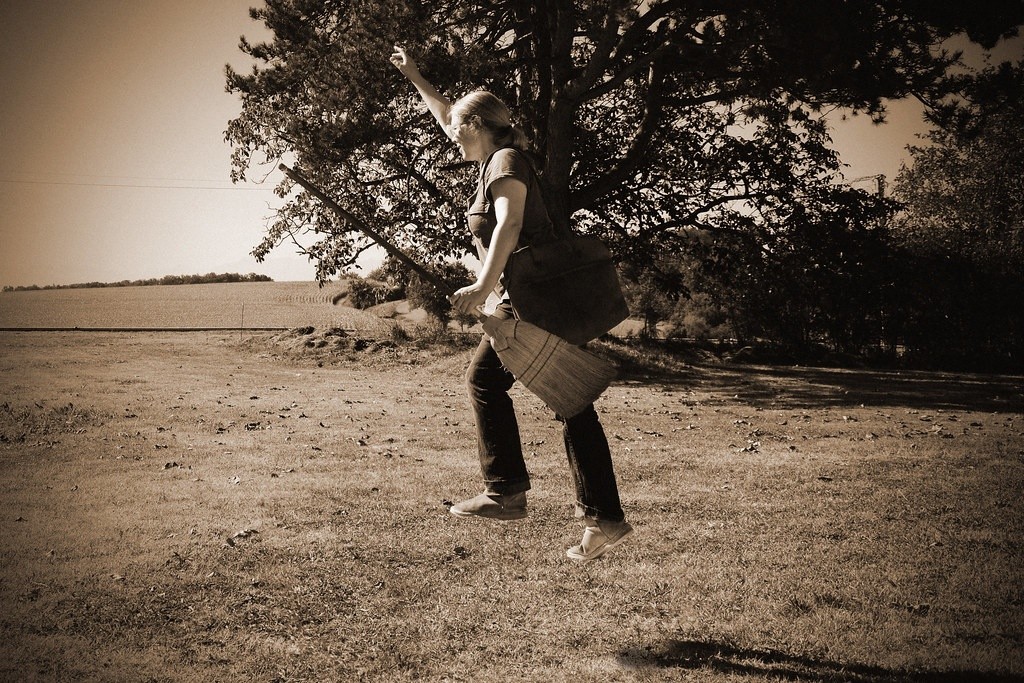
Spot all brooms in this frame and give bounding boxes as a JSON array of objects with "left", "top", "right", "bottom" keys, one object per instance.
[{"left": 277, "top": 163, "right": 619, "bottom": 419}]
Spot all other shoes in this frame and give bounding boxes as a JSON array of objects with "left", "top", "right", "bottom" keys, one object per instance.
[
  {"left": 448, "top": 486, "right": 528, "bottom": 521},
  {"left": 567, "top": 516, "right": 635, "bottom": 560}
]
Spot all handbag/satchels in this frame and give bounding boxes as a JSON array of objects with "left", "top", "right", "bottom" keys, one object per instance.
[{"left": 503, "top": 233, "right": 629, "bottom": 346}]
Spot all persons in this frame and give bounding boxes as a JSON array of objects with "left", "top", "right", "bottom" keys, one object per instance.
[{"left": 388, "top": 45, "right": 635, "bottom": 560}]
